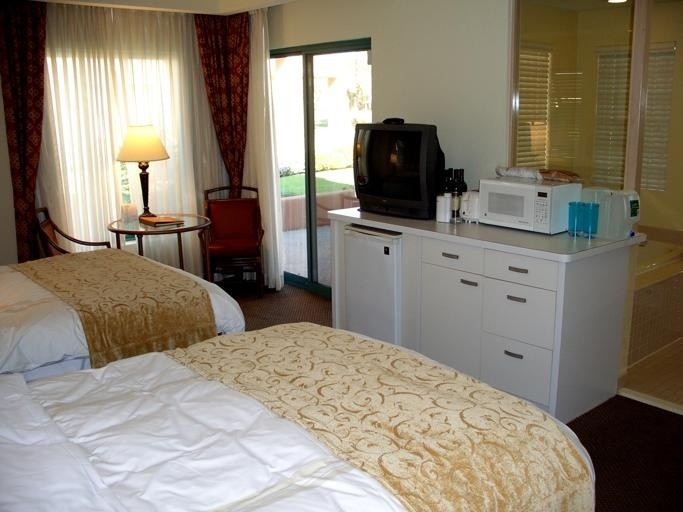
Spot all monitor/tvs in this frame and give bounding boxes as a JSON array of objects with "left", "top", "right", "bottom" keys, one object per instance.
[{"left": 353, "top": 124, "right": 445, "bottom": 220}]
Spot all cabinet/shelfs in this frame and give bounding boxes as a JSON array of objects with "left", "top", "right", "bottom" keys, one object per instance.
[{"left": 328, "top": 205, "right": 648, "bottom": 425}]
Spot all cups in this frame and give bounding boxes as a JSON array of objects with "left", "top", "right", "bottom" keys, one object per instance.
[{"left": 568, "top": 201, "right": 599, "bottom": 238}]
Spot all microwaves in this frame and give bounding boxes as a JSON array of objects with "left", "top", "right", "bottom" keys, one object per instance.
[{"left": 477, "top": 177, "right": 583, "bottom": 236}]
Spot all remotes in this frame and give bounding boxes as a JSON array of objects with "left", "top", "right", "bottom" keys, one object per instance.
[{"left": 383, "top": 118, "right": 404, "bottom": 124}]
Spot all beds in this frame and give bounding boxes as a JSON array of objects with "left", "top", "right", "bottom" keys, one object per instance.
[
  {"left": 1, "top": 323, "right": 598, "bottom": 510},
  {"left": 0, "top": 251, "right": 248, "bottom": 382}
]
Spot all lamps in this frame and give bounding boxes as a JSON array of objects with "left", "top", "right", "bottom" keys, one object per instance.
[{"left": 116, "top": 124, "right": 170, "bottom": 221}]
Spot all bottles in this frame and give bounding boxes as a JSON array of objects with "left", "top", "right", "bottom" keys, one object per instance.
[{"left": 445, "top": 167, "right": 467, "bottom": 223}]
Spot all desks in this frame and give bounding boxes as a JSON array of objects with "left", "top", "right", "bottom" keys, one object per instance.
[{"left": 107, "top": 213, "right": 211, "bottom": 282}]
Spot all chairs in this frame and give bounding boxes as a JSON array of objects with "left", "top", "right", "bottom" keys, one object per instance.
[
  {"left": 198, "top": 185, "right": 264, "bottom": 298},
  {"left": 34, "top": 206, "right": 110, "bottom": 261}
]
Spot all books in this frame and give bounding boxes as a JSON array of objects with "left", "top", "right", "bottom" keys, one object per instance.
[{"left": 137, "top": 216, "right": 184, "bottom": 228}]
